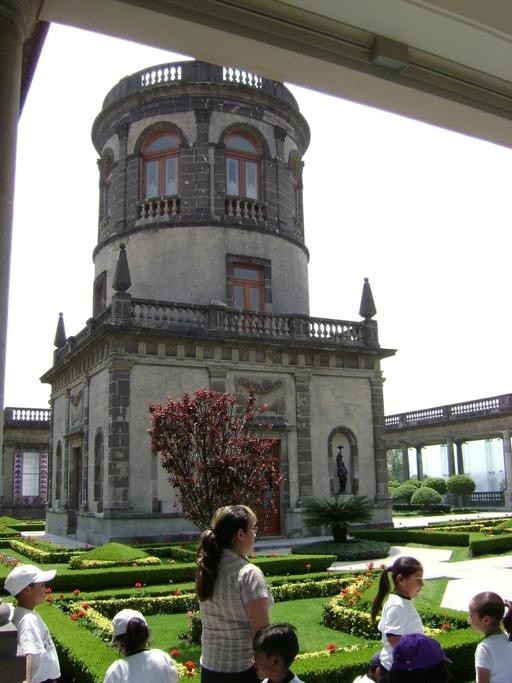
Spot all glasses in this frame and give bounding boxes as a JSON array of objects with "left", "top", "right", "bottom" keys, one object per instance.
[{"left": 247, "top": 526, "right": 258, "bottom": 533}]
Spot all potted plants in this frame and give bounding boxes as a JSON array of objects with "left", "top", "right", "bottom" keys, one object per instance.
[{"left": 297, "top": 493, "right": 375, "bottom": 542}]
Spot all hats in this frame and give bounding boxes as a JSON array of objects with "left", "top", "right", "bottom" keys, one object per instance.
[
  {"left": 370, "top": 651, "right": 381, "bottom": 665},
  {"left": 392, "top": 632, "right": 453, "bottom": 670},
  {"left": 4, "top": 564, "right": 57, "bottom": 596},
  {"left": 109, "top": 608, "right": 147, "bottom": 645}
]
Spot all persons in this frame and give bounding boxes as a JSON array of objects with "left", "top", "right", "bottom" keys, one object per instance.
[
  {"left": 353, "top": 652, "right": 382, "bottom": 683},
  {"left": 370, "top": 555, "right": 425, "bottom": 683},
  {"left": 336, "top": 453, "right": 349, "bottom": 495},
  {"left": 386, "top": 632, "right": 456, "bottom": 683},
  {"left": 196, "top": 504, "right": 275, "bottom": 683},
  {"left": 103, "top": 608, "right": 179, "bottom": 683},
  {"left": 4, "top": 564, "right": 61, "bottom": 683},
  {"left": 469, "top": 591, "right": 512, "bottom": 683},
  {"left": 253, "top": 622, "right": 303, "bottom": 683}
]
